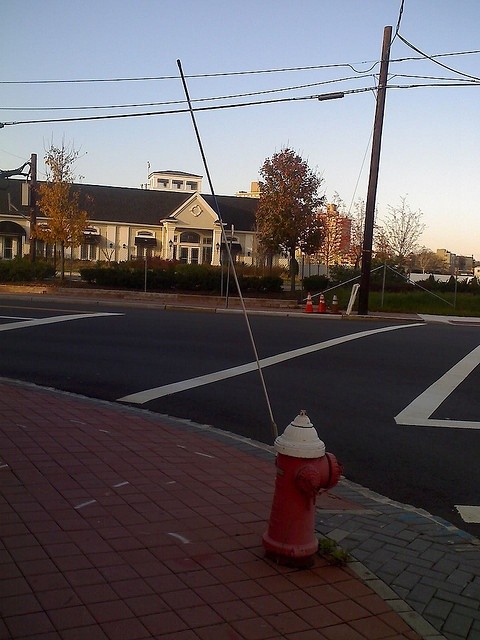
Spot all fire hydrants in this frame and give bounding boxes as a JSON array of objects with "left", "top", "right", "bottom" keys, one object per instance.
[{"left": 261, "top": 408, "right": 345, "bottom": 561}]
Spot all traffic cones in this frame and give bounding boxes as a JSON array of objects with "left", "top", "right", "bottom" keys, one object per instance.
[
  {"left": 305, "top": 291, "right": 314, "bottom": 313},
  {"left": 331, "top": 293, "right": 340, "bottom": 313},
  {"left": 317, "top": 294, "right": 327, "bottom": 313}
]
